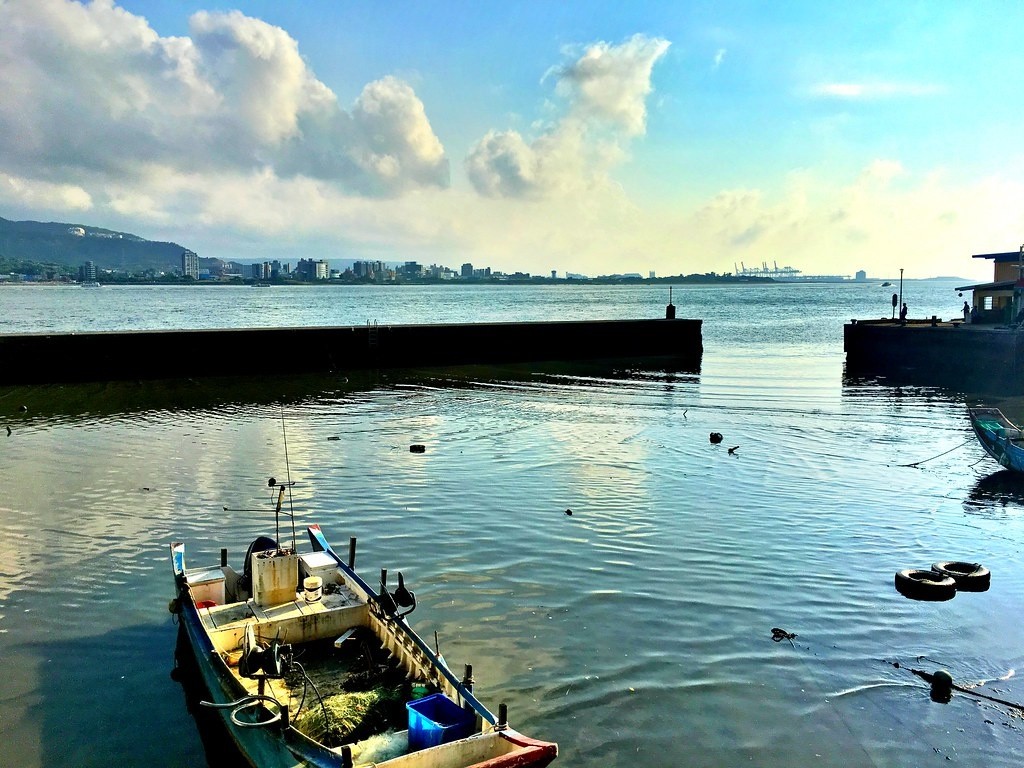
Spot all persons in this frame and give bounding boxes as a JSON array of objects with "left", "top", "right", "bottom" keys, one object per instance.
[
  {"left": 901, "top": 303, "right": 907, "bottom": 327},
  {"left": 971, "top": 305, "right": 977, "bottom": 314},
  {"left": 964, "top": 302, "right": 969, "bottom": 318}
]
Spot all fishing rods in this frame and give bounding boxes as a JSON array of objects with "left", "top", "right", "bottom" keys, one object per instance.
[{"left": 280, "top": 404, "right": 301, "bottom": 554}]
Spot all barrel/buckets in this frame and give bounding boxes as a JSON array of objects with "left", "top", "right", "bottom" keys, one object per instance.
[
  {"left": 196, "top": 600, "right": 218, "bottom": 609},
  {"left": 303, "top": 576, "right": 323, "bottom": 604}
]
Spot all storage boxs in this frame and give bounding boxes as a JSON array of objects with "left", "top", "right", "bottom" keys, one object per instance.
[
  {"left": 186, "top": 569, "right": 226, "bottom": 605},
  {"left": 405, "top": 693, "right": 477, "bottom": 746}
]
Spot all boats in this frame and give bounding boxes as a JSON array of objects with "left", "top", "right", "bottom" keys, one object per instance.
[
  {"left": 82, "top": 281, "right": 101, "bottom": 287},
  {"left": 882, "top": 282, "right": 891, "bottom": 286},
  {"left": 969, "top": 408, "right": 1024, "bottom": 479},
  {"left": 169, "top": 409, "right": 559, "bottom": 768},
  {"left": 251, "top": 283, "right": 271, "bottom": 287}
]
